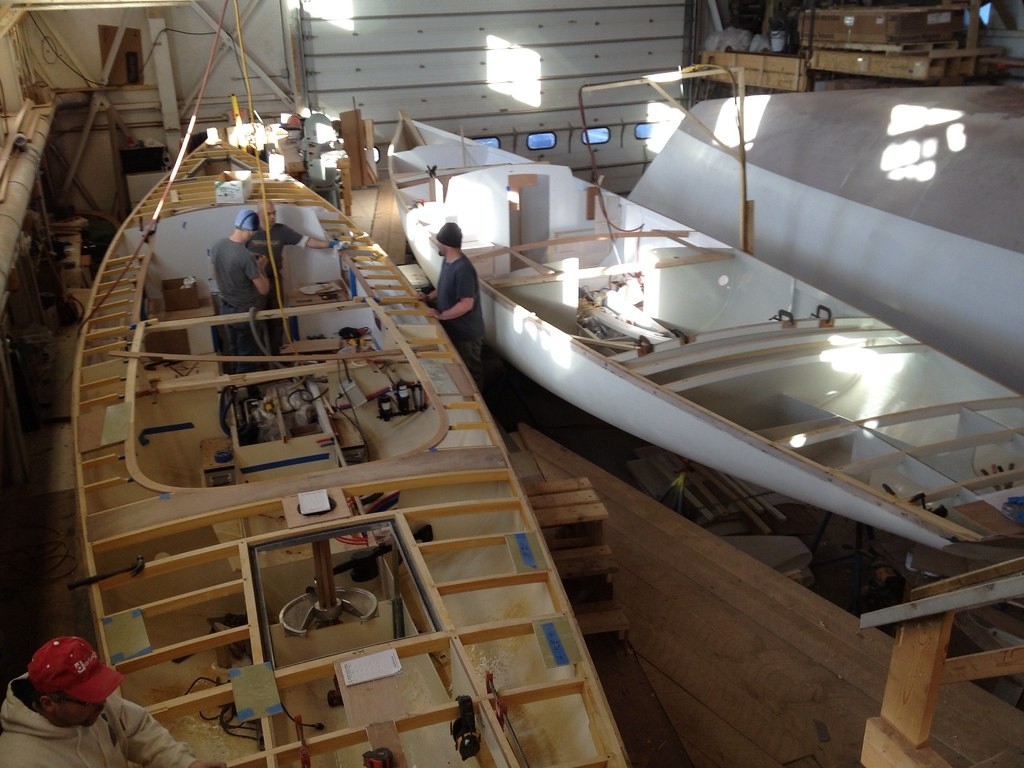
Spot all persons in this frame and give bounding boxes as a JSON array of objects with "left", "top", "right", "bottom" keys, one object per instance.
[
  {"left": 0, "top": 634, "right": 228, "bottom": 768},
  {"left": 209, "top": 208, "right": 270, "bottom": 374},
  {"left": 415, "top": 222, "right": 491, "bottom": 384},
  {"left": 246, "top": 199, "right": 347, "bottom": 354}
]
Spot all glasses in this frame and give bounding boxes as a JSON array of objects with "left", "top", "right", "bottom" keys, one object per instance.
[{"left": 52, "top": 692, "right": 90, "bottom": 707}]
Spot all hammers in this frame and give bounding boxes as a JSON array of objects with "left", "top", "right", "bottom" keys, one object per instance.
[{"left": 67, "top": 555, "right": 146, "bottom": 590}]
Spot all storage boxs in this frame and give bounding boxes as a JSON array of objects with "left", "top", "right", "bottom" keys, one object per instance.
[
  {"left": 226, "top": 109, "right": 382, "bottom": 191},
  {"left": 798, "top": 3, "right": 965, "bottom": 42},
  {"left": 161, "top": 276, "right": 201, "bottom": 310},
  {"left": 214, "top": 169, "right": 252, "bottom": 203}
]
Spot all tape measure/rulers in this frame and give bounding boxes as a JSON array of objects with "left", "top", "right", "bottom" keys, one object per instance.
[{"left": 362, "top": 746, "right": 392, "bottom": 768}]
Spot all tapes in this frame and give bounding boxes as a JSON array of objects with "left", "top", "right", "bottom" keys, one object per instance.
[{"left": 214, "top": 449, "right": 232, "bottom": 463}]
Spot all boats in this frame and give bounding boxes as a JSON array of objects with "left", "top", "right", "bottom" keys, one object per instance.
[
  {"left": 65, "top": 127, "right": 631, "bottom": 768},
  {"left": 383, "top": 106, "right": 1024, "bottom": 570}
]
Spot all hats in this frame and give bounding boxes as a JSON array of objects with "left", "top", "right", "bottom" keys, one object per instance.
[
  {"left": 436, "top": 222, "right": 462, "bottom": 248},
  {"left": 28, "top": 635, "right": 128, "bottom": 703},
  {"left": 234, "top": 209, "right": 259, "bottom": 231}
]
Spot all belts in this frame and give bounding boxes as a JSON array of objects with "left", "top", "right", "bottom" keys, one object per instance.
[{"left": 222, "top": 299, "right": 233, "bottom": 309}]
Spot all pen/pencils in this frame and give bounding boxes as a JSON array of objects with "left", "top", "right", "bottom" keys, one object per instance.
[{"left": 296, "top": 299, "right": 313, "bottom": 303}]
